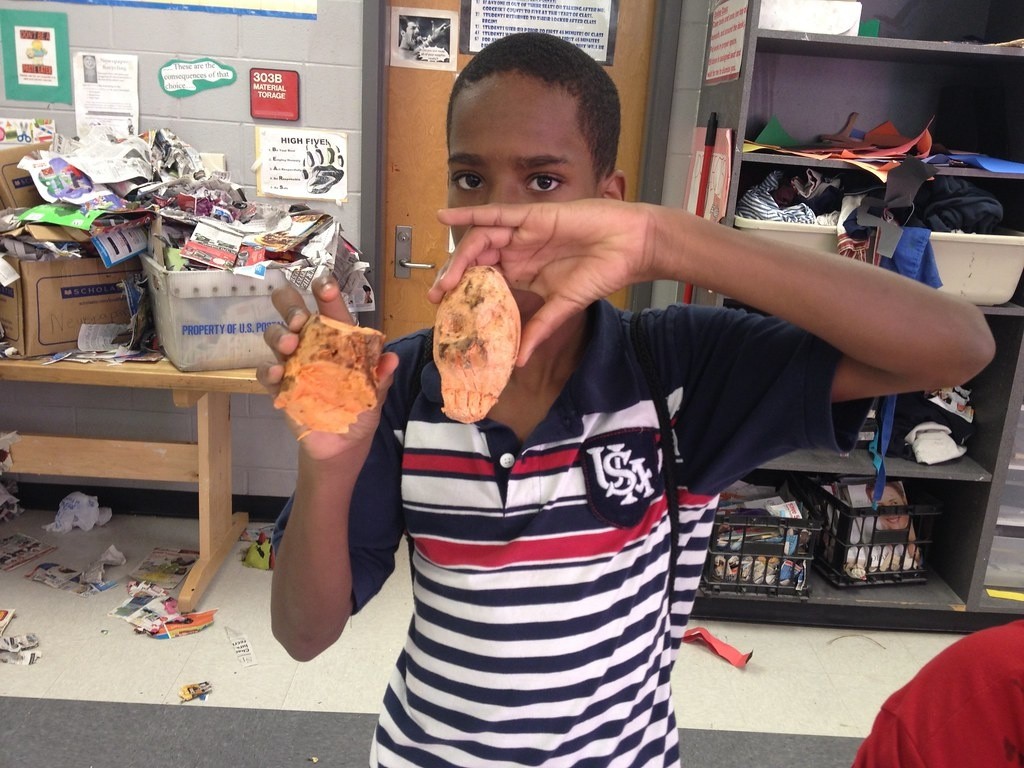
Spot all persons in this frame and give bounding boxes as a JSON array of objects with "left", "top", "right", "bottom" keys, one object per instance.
[
  {"left": 256, "top": 32, "right": 997, "bottom": 768},
  {"left": 865, "top": 481, "right": 920, "bottom": 563},
  {"left": 849, "top": 619, "right": 1024, "bottom": 768},
  {"left": 400, "top": 16, "right": 450, "bottom": 60}
]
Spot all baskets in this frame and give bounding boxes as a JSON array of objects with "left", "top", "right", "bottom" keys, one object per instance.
[
  {"left": 700, "top": 480, "right": 823, "bottom": 602},
  {"left": 796, "top": 482, "right": 951, "bottom": 589}
]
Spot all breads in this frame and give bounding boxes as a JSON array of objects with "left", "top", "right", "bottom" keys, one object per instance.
[
  {"left": 272, "top": 313, "right": 384, "bottom": 435},
  {"left": 432, "top": 264, "right": 521, "bottom": 423}
]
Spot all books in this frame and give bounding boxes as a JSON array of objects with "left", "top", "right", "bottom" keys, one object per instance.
[{"left": 819, "top": 476, "right": 919, "bottom": 572}]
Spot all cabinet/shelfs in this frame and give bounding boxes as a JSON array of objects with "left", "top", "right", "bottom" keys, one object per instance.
[{"left": 659, "top": 0, "right": 1024, "bottom": 632}]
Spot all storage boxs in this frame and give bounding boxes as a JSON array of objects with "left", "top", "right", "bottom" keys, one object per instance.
[
  {"left": 0, "top": 252, "right": 149, "bottom": 359},
  {"left": 138, "top": 251, "right": 320, "bottom": 371},
  {"left": 928, "top": 228, "right": 1024, "bottom": 306},
  {"left": 734, "top": 215, "right": 877, "bottom": 266},
  {"left": 791, "top": 469, "right": 946, "bottom": 589},
  {"left": 0, "top": 143, "right": 54, "bottom": 209},
  {"left": 700, "top": 475, "right": 827, "bottom": 600}
]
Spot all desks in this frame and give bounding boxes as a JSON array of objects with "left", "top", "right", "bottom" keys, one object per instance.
[{"left": 0, "top": 346, "right": 267, "bottom": 611}]
